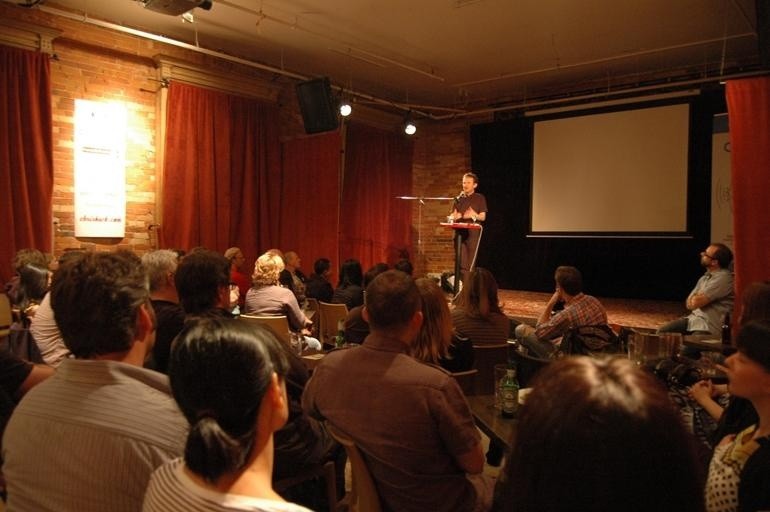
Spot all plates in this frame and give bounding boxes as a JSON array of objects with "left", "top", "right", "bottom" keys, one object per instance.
[{"left": 517, "top": 387, "right": 535, "bottom": 404}]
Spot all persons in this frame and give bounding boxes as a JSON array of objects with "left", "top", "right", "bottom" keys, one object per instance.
[
  {"left": 0, "top": 243, "right": 769, "bottom": 511},
  {"left": 448, "top": 173, "right": 487, "bottom": 304}
]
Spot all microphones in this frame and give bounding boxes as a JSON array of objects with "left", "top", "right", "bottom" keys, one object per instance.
[{"left": 456, "top": 191, "right": 464, "bottom": 199}]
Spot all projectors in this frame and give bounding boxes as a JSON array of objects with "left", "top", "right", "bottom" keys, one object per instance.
[{"left": 144, "top": 0, "right": 204, "bottom": 16}]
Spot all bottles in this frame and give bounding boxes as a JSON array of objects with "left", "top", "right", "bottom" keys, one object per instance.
[
  {"left": 500, "top": 368, "right": 521, "bottom": 418},
  {"left": 334, "top": 319, "right": 347, "bottom": 348},
  {"left": 674, "top": 345, "right": 713, "bottom": 374},
  {"left": 290, "top": 326, "right": 302, "bottom": 355},
  {"left": 720, "top": 311, "right": 732, "bottom": 345}
]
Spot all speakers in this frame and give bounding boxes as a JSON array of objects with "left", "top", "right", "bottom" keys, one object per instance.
[{"left": 296, "top": 77, "right": 338, "bottom": 134}]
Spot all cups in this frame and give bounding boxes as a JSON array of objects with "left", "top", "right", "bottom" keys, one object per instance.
[
  {"left": 342, "top": 342, "right": 360, "bottom": 349},
  {"left": 446, "top": 215, "right": 455, "bottom": 224},
  {"left": 494, "top": 364, "right": 515, "bottom": 408}
]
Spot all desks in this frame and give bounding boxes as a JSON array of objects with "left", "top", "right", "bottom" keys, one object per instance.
[{"left": 683, "top": 335, "right": 738, "bottom": 353}]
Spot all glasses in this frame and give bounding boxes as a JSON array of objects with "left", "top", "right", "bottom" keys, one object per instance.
[{"left": 703, "top": 251, "right": 714, "bottom": 260}]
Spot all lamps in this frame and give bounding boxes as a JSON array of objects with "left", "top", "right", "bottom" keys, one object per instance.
[
  {"left": 334, "top": 86, "right": 351, "bottom": 117},
  {"left": 401, "top": 109, "right": 417, "bottom": 136}
]
